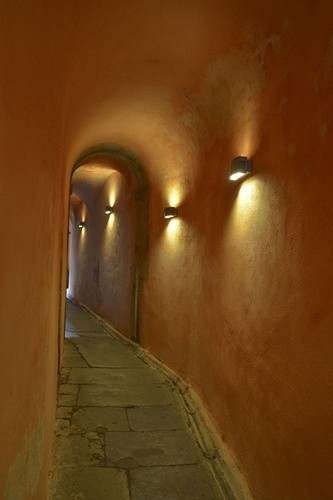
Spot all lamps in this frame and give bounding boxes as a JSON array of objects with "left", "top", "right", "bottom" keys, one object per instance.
[
  {"left": 229, "top": 156, "right": 252, "bottom": 181},
  {"left": 164, "top": 207, "right": 178, "bottom": 219},
  {"left": 105, "top": 206, "right": 113, "bottom": 215},
  {"left": 79, "top": 222, "right": 85, "bottom": 228}
]
